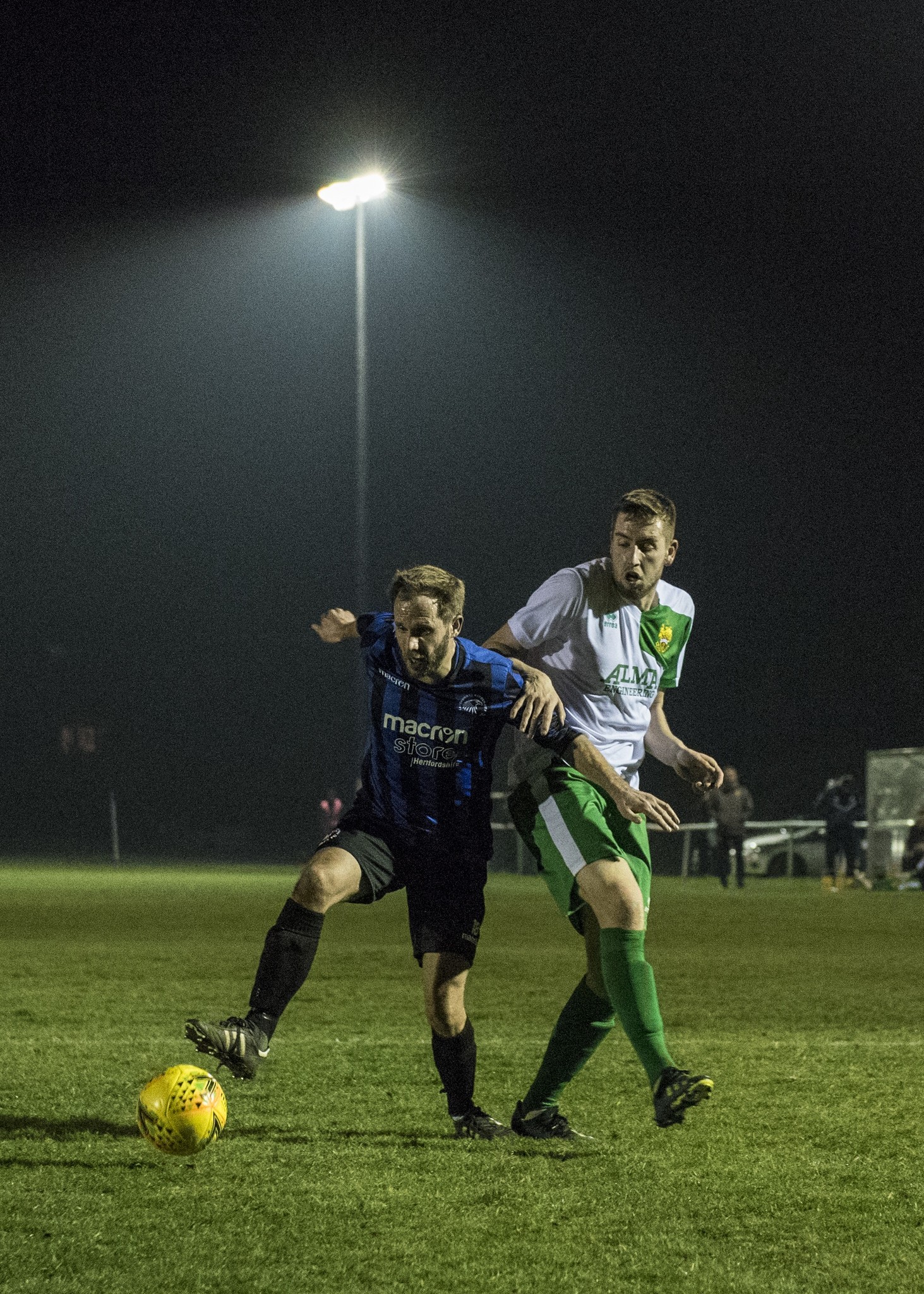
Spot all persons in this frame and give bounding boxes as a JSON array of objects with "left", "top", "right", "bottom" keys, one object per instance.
[
  {"left": 185, "top": 566, "right": 681, "bottom": 1140},
  {"left": 797, "top": 774, "right": 873, "bottom": 891},
  {"left": 894, "top": 807, "right": 923, "bottom": 891},
  {"left": 318, "top": 786, "right": 345, "bottom": 839},
  {"left": 480, "top": 489, "right": 724, "bottom": 1139},
  {"left": 703, "top": 764, "right": 754, "bottom": 891}
]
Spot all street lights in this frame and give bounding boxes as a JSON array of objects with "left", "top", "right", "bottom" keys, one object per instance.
[{"left": 317, "top": 169, "right": 389, "bottom": 616}]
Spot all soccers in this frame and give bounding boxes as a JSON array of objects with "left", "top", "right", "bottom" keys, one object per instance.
[{"left": 136, "top": 1063, "right": 228, "bottom": 1157}]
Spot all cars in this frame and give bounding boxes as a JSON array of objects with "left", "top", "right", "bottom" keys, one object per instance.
[{"left": 729, "top": 811, "right": 908, "bottom": 878}]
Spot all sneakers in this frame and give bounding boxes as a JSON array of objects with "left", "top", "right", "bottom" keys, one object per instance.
[
  {"left": 509, "top": 1101, "right": 594, "bottom": 1141},
  {"left": 452, "top": 1107, "right": 512, "bottom": 1139},
  {"left": 184, "top": 1016, "right": 271, "bottom": 1081},
  {"left": 651, "top": 1065, "right": 714, "bottom": 1127}
]
